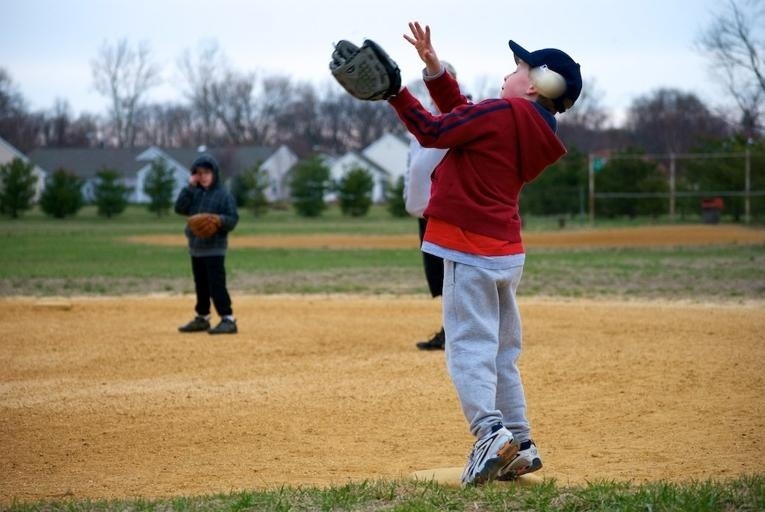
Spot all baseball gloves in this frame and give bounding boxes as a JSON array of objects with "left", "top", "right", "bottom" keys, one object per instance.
[
  {"left": 187, "top": 212, "right": 221, "bottom": 238},
  {"left": 329, "top": 38, "right": 403, "bottom": 103}
]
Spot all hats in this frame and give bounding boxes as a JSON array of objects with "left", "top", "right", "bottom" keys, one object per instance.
[{"left": 508, "top": 39, "right": 582, "bottom": 114}]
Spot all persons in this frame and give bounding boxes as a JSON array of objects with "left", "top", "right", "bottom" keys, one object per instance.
[
  {"left": 174, "top": 154, "right": 239, "bottom": 334},
  {"left": 330, "top": 21, "right": 583, "bottom": 489},
  {"left": 402, "top": 61, "right": 473, "bottom": 350}
]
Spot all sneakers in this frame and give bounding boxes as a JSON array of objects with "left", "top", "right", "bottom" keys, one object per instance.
[
  {"left": 206, "top": 317, "right": 239, "bottom": 335},
  {"left": 415, "top": 327, "right": 445, "bottom": 352},
  {"left": 489, "top": 436, "right": 543, "bottom": 480},
  {"left": 459, "top": 421, "right": 517, "bottom": 493},
  {"left": 177, "top": 315, "right": 212, "bottom": 333}
]
